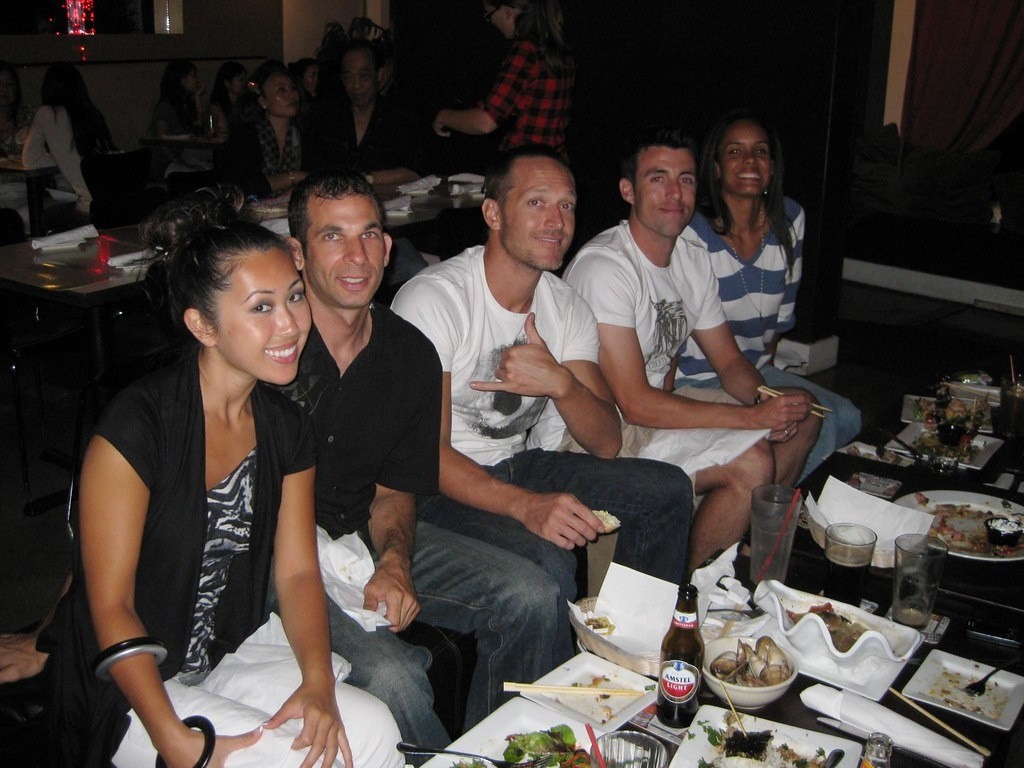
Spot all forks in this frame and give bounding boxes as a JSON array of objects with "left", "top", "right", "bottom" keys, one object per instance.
[
  {"left": 396, "top": 740, "right": 552, "bottom": 768},
  {"left": 961, "top": 656, "right": 1024, "bottom": 698}
]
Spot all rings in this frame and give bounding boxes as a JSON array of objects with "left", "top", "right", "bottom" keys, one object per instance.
[{"left": 785, "top": 429, "right": 789, "bottom": 437}]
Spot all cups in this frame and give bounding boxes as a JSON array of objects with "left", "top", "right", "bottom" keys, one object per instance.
[
  {"left": 749, "top": 483, "right": 802, "bottom": 586},
  {"left": 590, "top": 730, "right": 669, "bottom": 768},
  {"left": 206, "top": 112, "right": 217, "bottom": 133},
  {"left": 998, "top": 371, "right": 1024, "bottom": 443},
  {"left": 823, "top": 522, "right": 877, "bottom": 609},
  {"left": 892, "top": 533, "right": 948, "bottom": 631}
]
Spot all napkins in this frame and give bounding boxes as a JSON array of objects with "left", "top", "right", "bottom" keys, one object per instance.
[{"left": 798, "top": 681, "right": 986, "bottom": 768}]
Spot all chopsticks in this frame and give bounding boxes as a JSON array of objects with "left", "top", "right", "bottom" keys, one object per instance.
[
  {"left": 943, "top": 381, "right": 1000, "bottom": 401},
  {"left": 888, "top": 687, "right": 991, "bottom": 757},
  {"left": 756, "top": 385, "right": 833, "bottom": 419},
  {"left": 503, "top": 682, "right": 643, "bottom": 696}
]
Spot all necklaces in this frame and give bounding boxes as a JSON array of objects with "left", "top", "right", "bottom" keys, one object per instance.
[{"left": 724, "top": 208, "right": 764, "bottom": 319}]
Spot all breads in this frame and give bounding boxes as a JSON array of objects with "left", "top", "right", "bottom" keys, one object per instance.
[{"left": 591, "top": 510, "right": 621, "bottom": 533}]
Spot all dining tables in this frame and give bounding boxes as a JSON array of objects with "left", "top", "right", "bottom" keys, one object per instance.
[
  {"left": 754, "top": 368, "right": 1024, "bottom": 619},
  {"left": 413, "top": 603, "right": 1024, "bottom": 768}
]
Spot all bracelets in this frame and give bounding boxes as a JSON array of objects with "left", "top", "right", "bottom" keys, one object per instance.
[
  {"left": 91, "top": 637, "right": 168, "bottom": 681},
  {"left": 290, "top": 171, "right": 295, "bottom": 187},
  {"left": 752, "top": 393, "right": 761, "bottom": 406},
  {"left": 155, "top": 716, "right": 215, "bottom": 768}
]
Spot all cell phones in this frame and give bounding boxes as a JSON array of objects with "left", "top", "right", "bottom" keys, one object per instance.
[{"left": 966, "top": 619, "right": 1023, "bottom": 648}]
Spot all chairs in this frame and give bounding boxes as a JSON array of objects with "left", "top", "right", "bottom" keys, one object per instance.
[{"left": 0, "top": 138, "right": 244, "bottom": 562}]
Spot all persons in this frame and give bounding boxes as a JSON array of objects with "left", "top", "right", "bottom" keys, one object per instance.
[
  {"left": 0, "top": 170, "right": 577, "bottom": 768},
  {"left": 389, "top": 142, "right": 695, "bottom": 668},
  {"left": 685, "top": 111, "right": 863, "bottom": 487},
  {"left": 0, "top": 0, "right": 593, "bottom": 325},
  {"left": 50, "top": 180, "right": 408, "bottom": 768},
  {"left": 560, "top": 118, "right": 822, "bottom": 568}
]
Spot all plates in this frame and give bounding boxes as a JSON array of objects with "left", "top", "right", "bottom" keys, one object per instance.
[
  {"left": 750, "top": 599, "right": 926, "bottom": 702},
  {"left": 669, "top": 705, "right": 863, "bottom": 768},
  {"left": 885, "top": 421, "right": 1004, "bottom": 471},
  {"left": 893, "top": 490, "right": 1024, "bottom": 562},
  {"left": 949, "top": 381, "right": 1001, "bottom": 408},
  {"left": 901, "top": 648, "right": 1024, "bottom": 731},
  {"left": 520, "top": 651, "right": 659, "bottom": 734},
  {"left": 901, "top": 395, "right": 994, "bottom": 434}
]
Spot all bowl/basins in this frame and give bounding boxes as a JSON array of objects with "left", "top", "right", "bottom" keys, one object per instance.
[
  {"left": 753, "top": 579, "right": 921, "bottom": 669},
  {"left": 701, "top": 635, "right": 799, "bottom": 710}
]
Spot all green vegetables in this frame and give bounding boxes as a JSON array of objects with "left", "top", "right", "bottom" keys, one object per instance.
[
  {"left": 502, "top": 724, "right": 585, "bottom": 768},
  {"left": 687, "top": 719, "right": 727, "bottom": 768}
]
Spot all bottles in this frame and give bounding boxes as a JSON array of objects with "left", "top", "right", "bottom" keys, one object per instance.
[
  {"left": 656, "top": 584, "right": 705, "bottom": 728},
  {"left": 859, "top": 731, "right": 893, "bottom": 768}
]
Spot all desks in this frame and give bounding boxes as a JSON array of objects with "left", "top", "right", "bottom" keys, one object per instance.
[{"left": 0, "top": 172, "right": 491, "bottom": 497}]
